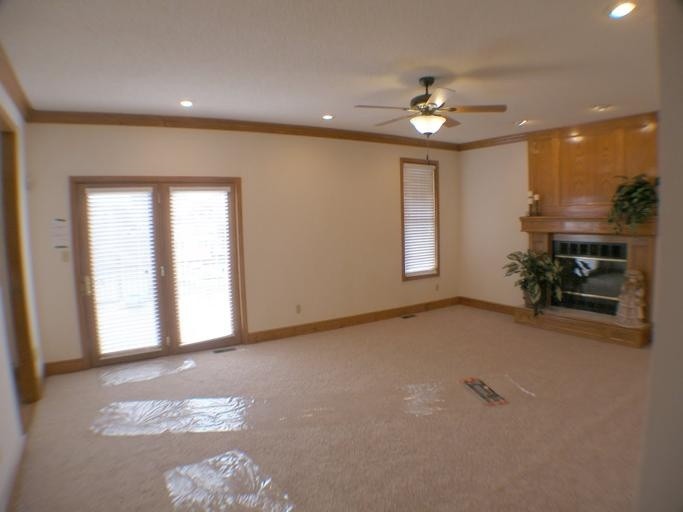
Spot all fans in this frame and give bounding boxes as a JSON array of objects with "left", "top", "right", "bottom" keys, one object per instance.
[{"left": 355, "top": 74, "right": 507, "bottom": 128}]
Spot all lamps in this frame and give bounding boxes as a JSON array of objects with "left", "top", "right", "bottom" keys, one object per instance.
[{"left": 410, "top": 75, "right": 446, "bottom": 138}]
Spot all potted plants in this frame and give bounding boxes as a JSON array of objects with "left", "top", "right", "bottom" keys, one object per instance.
[{"left": 507, "top": 252, "right": 563, "bottom": 319}]
